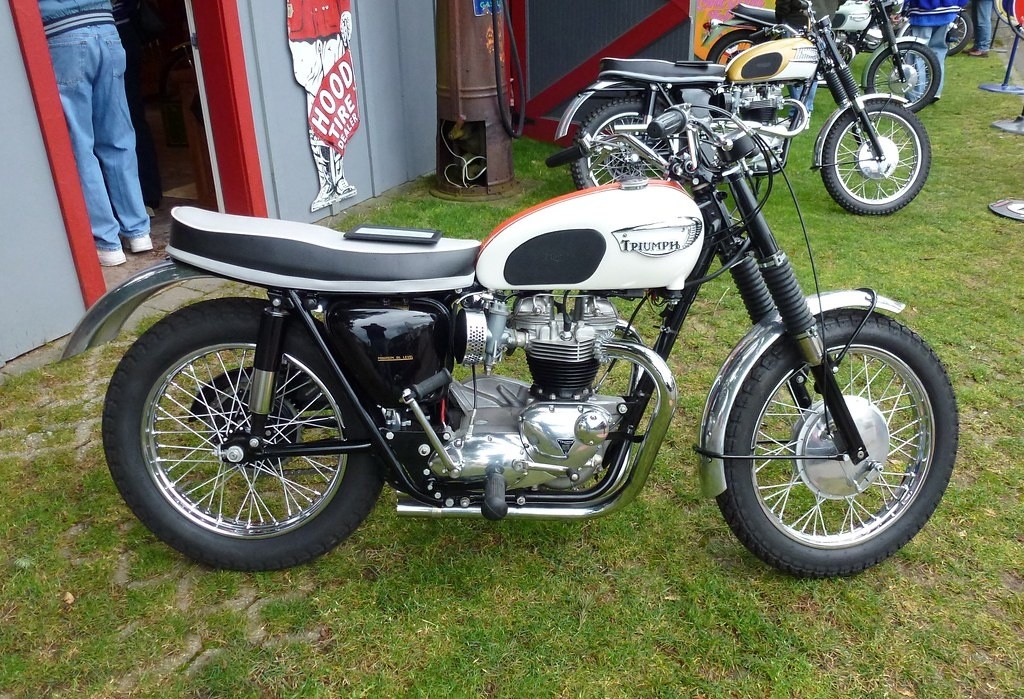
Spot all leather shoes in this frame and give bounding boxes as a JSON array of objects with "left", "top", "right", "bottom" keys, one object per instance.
[{"left": 962, "top": 47, "right": 989, "bottom": 56}]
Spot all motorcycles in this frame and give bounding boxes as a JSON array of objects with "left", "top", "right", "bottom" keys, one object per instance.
[{"left": 56, "top": 0, "right": 978, "bottom": 582}]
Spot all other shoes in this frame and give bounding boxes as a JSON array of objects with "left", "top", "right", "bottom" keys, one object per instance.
[{"left": 145, "top": 206, "right": 155, "bottom": 217}]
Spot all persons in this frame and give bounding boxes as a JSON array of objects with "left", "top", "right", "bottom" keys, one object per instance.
[
  {"left": 962, "top": 0, "right": 992, "bottom": 55},
  {"left": 38, "top": 0, "right": 163, "bottom": 266},
  {"left": 901, "top": 0, "right": 970, "bottom": 104}
]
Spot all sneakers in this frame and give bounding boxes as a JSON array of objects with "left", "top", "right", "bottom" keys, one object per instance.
[
  {"left": 96, "top": 245, "right": 126, "bottom": 267},
  {"left": 120, "top": 232, "right": 153, "bottom": 252}
]
